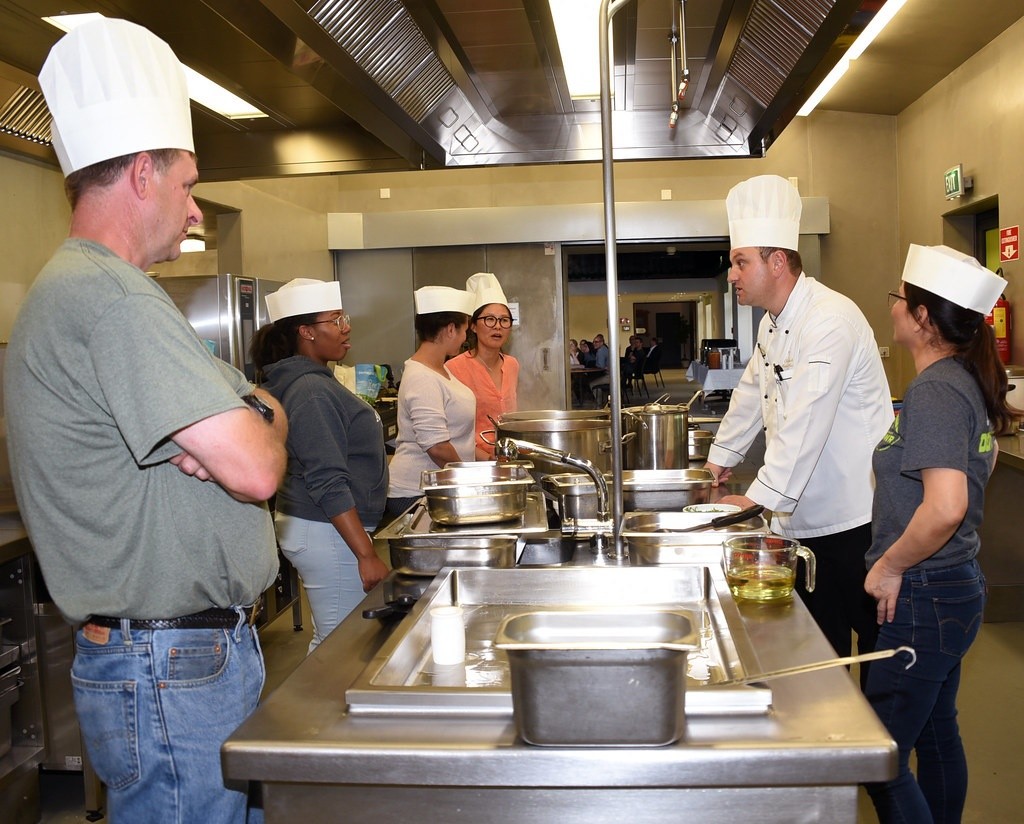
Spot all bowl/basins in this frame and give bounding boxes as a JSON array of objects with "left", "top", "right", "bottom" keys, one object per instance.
[{"left": 683, "top": 504, "right": 742, "bottom": 512}]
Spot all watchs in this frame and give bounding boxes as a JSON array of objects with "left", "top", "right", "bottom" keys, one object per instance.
[{"left": 241, "top": 392, "right": 274, "bottom": 421}]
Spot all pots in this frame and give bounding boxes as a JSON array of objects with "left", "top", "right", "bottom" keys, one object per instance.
[
  {"left": 1004, "top": 364, "right": 1024, "bottom": 411},
  {"left": 479, "top": 398, "right": 694, "bottom": 491},
  {"left": 686, "top": 430, "right": 714, "bottom": 460}
]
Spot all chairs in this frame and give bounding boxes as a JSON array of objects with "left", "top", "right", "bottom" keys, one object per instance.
[{"left": 590, "top": 345, "right": 666, "bottom": 411}]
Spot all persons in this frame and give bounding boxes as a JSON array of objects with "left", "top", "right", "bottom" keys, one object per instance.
[
  {"left": 704, "top": 174, "right": 894, "bottom": 669},
  {"left": 569, "top": 334, "right": 662, "bottom": 409},
  {"left": 4, "top": 19, "right": 287, "bottom": 823},
  {"left": 861, "top": 243, "right": 1024, "bottom": 824},
  {"left": 443, "top": 272, "right": 518, "bottom": 462},
  {"left": 388, "top": 286, "right": 477, "bottom": 521},
  {"left": 249, "top": 277, "right": 389, "bottom": 659}
]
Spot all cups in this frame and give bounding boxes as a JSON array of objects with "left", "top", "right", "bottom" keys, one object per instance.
[
  {"left": 721, "top": 535, "right": 817, "bottom": 601},
  {"left": 429, "top": 606, "right": 465, "bottom": 665},
  {"left": 431, "top": 663, "right": 466, "bottom": 687}
]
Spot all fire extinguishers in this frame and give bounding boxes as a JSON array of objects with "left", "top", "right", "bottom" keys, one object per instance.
[{"left": 982, "top": 266, "right": 1012, "bottom": 365}]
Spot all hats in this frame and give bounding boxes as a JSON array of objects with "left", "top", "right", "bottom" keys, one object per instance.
[
  {"left": 901, "top": 243, "right": 1008, "bottom": 316},
  {"left": 36, "top": 18, "right": 195, "bottom": 179},
  {"left": 265, "top": 278, "right": 343, "bottom": 323},
  {"left": 466, "top": 273, "right": 510, "bottom": 316},
  {"left": 725, "top": 175, "right": 802, "bottom": 252},
  {"left": 414, "top": 286, "right": 475, "bottom": 317}
]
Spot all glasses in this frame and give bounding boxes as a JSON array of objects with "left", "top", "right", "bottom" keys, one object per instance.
[
  {"left": 476, "top": 316, "right": 514, "bottom": 328},
  {"left": 308, "top": 315, "right": 351, "bottom": 332},
  {"left": 593, "top": 341, "right": 602, "bottom": 343},
  {"left": 887, "top": 291, "right": 908, "bottom": 306}
]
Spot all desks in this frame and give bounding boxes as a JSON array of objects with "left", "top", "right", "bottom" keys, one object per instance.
[
  {"left": 571, "top": 367, "right": 607, "bottom": 408},
  {"left": 685, "top": 361, "right": 745, "bottom": 408}
]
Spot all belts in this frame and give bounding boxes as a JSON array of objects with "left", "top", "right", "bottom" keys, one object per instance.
[{"left": 86, "top": 596, "right": 265, "bottom": 629}]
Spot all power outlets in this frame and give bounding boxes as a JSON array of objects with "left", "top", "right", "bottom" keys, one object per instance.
[{"left": 879, "top": 347, "right": 889, "bottom": 357}]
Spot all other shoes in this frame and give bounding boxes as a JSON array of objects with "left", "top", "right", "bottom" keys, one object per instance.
[{"left": 593, "top": 404, "right": 604, "bottom": 410}]
